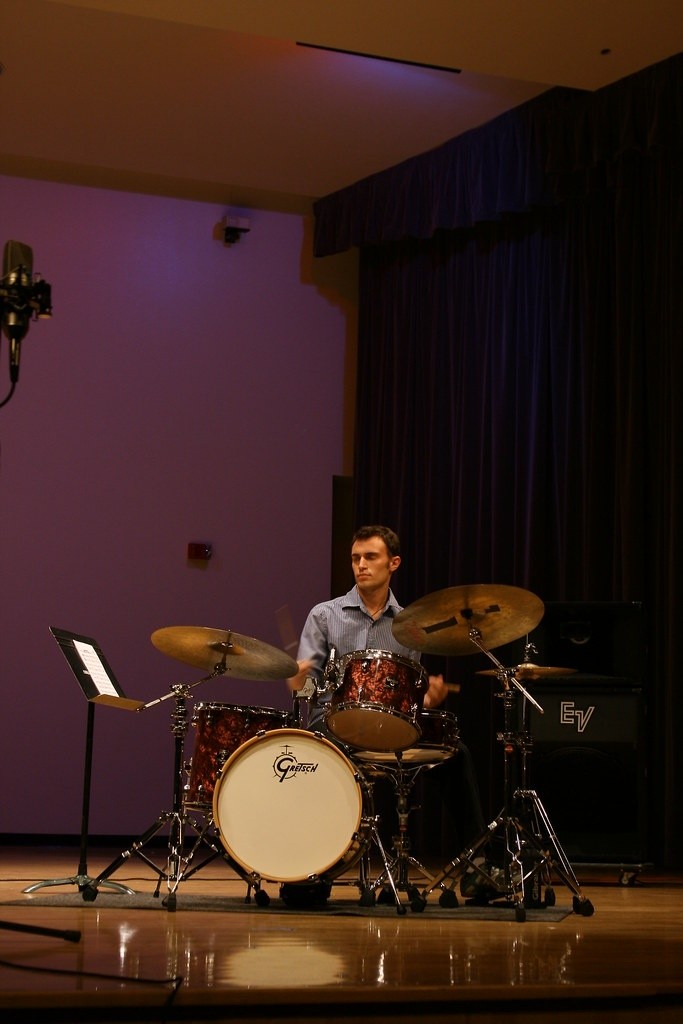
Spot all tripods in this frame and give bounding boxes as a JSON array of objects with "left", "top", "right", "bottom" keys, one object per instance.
[
  {"left": 357, "top": 625, "right": 593, "bottom": 924},
  {"left": 22, "top": 627, "right": 232, "bottom": 913}
]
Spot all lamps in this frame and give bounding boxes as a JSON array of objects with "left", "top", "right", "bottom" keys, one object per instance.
[{"left": 223, "top": 215, "right": 250, "bottom": 243}]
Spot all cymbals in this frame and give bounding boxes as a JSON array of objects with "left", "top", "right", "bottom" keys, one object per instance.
[
  {"left": 473, "top": 665, "right": 577, "bottom": 681},
  {"left": 149, "top": 624, "right": 299, "bottom": 682},
  {"left": 392, "top": 584, "right": 544, "bottom": 658}
]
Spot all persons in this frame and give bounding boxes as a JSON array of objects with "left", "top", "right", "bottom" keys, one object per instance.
[{"left": 278, "top": 526, "right": 525, "bottom": 904}]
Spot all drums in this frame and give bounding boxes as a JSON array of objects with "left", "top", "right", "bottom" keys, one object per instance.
[
  {"left": 180, "top": 701, "right": 299, "bottom": 823},
  {"left": 211, "top": 725, "right": 377, "bottom": 882},
  {"left": 345, "top": 707, "right": 458, "bottom": 766},
  {"left": 328, "top": 646, "right": 422, "bottom": 750}
]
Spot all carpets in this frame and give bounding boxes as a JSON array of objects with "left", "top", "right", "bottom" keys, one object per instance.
[{"left": 0, "top": 894, "right": 576, "bottom": 924}]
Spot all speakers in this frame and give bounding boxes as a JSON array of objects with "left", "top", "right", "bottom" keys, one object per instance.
[{"left": 505, "top": 682, "right": 648, "bottom": 866}]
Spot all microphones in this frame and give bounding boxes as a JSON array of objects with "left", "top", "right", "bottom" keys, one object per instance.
[{"left": 2, "top": 240, "right": 33, "bottom": 384}]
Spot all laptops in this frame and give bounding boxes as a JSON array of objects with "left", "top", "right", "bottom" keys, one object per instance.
[{"left": 527, "top": 603, "right": 644, "bottom": 688}]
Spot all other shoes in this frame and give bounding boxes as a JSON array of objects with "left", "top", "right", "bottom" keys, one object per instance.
[
  {"left": 460, "top": 863, "right": 522, "bottom": 896},
  {"left": 282, "top": 881, "right": 330, "bottom": 910}
]
